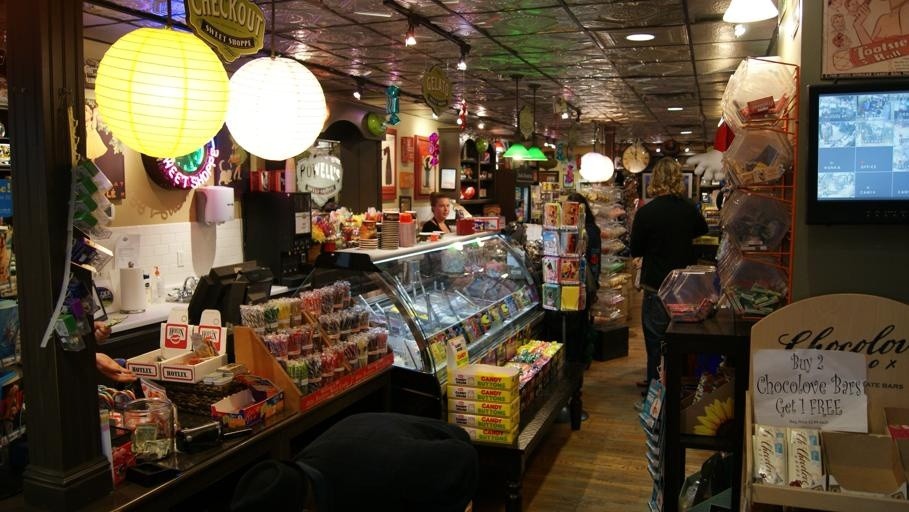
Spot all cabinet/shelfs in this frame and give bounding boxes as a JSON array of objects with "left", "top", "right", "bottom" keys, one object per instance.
[
  {"left": 541, "top": 226, "right": 590, "bottom": 314},
  {"left": 438, "top": 127, "right": 495, "bottom": 205},
  {"left": 722, "top": 54, "right": 801, "bottom": 322},
  {"left": 292, "top": 230, "right": 545, "bottom": 401},
  {"left": 0, "top": 44, "right": 27, "bottom": 449}
]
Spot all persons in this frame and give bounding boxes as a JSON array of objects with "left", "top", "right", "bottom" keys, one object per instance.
[
  {"left": 417, "top": 189, "right": 455, "bottom": 287},
  {"left": 93, "top": 317, "right": 141, "bottom": 392},
  {"left": 647, "top": 387, "right": 664, "bottom": 419},
  {"left": 562, "top": 191, "right": 601, "bottom": 368},
  {"left": 628, "top": 154, "right": 713, "bottom": 397}
]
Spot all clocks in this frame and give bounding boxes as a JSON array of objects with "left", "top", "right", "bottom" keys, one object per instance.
[{"left": 621, "top": 143, "right": 651, "bottom": 174}]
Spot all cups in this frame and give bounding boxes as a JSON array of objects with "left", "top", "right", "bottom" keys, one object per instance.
[{"left": 123, "top": 397, "right": 175, "bottom": 463}]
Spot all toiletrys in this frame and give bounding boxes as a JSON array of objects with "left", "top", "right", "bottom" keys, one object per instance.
[{"left": 151, "top": 264, "right": 165, "bottom": 301}]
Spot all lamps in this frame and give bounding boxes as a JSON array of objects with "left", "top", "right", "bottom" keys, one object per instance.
[
  {"left": 527, "top": 83, "right": 549, "bottom": 162},
  {"left": 723, "top": 0, "right": 779, "bottom": 24},
  {"left": 404, "top": 14, "right": 471, "bottom": 71},
  {"left": 503, "top": 74, "right": 531, "bottom": 161}
]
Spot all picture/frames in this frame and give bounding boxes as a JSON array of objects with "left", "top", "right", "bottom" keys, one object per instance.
[
  {"left": 381, "top": 129, "right": 398, "bottom": 202},
  {"left": 414, "top": 135, "right": 436, "bottom": 201}
]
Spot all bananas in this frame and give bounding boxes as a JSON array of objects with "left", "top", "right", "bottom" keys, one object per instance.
[{"left": 694, "top": 397, "right": 734, "bottom": 436}]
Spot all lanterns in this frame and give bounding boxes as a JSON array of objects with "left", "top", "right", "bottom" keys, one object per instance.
[
  {"left": 225, "top": 55, "right": 328, "bottom": 163},
  {"left": 93, "top": 24, "right": 232, "bottom": 159}
]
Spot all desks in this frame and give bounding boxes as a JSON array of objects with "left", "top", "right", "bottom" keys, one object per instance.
[
  {"left": 107, "top": 300, "right": 190, "bottom": 333},
  {"left": 0, "top": 364, "right": 395, "bottom": 511},
  {"left": 661, "top": 308, "right": 754, "bottom": 512}
]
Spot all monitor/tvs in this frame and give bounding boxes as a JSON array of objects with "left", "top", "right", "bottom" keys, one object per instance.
[
  {"left": 187, "top": 260, "right": 273, "bottom": 327},
  {"left": 805, "top": 80, "right": 909, "bottom": 225}
]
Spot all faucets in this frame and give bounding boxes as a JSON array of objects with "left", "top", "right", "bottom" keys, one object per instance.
[{"left": 183, "top": 275, "right": 199, "bottom": 291}]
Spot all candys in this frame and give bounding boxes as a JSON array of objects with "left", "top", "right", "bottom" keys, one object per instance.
[
  {"left": 240, "top": 282, "right": 388, "bottom": 394},
  {"left": 98, "top": 385, "right": 136, "bottom": 414}
]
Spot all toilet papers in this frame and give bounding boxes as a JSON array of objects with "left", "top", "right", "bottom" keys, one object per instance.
[{"left": 119, "top": 267, "right": 147, "bottom": 311}]
